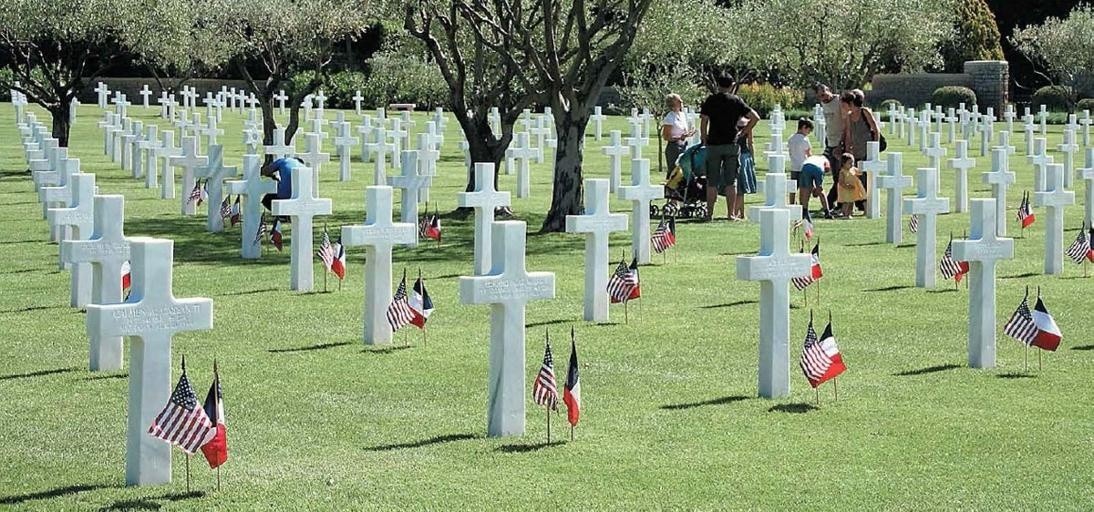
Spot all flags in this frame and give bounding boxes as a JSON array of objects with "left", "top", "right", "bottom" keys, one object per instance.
[
  {"left": 386, "top": 276, "right": 435, "bottom": 333},
  {"left": 909, "top": 193, "right": 1094, "bottom": 352},
  {"left": 252, "top": 210, "right": 283, "bottom": 253},
  {"left": 187, "top": 178, "right": 208, "bottom": 205},
  {"left": 799, "top": 322, "right": 847, "bottom": 388},
  {"left": 802, "top": 214, "right": 814, "bottom": 238},
  {"left": 220, "top": 194, "right": 240, "bottom": 226},
  {"left": 532, "top": 344, "right": 560, "bottom": 416},
  {"left": 651, "top": 216, "right": 676, "bottom": 253},
  {"left": 563, "top": 343, "right": 582, "bottom": 426},
  {"left": 317, "top": 232, "right": 346, "bottom": 280},
  {"left": 792, "top": 244, "right": 823, "bottom": 292},
  {"left": 200, "top": 375, "right": 228, "bottom": 469},
  {"left": 606, "top": 259, "right": 640, "bottom": 303},
  {"left": 419, "top": 212, "right": 441, "bottom": 241},
  {"left": 146, "top": 373, "right": 213, "bottom": 456}
]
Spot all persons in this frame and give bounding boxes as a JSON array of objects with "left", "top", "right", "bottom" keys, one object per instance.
[
  {"left": 261, "top": 158, "right": 306, "bottom": 223},
  {"left": 661, "top": 93, "right": 696, "bottom": 179},
  {"left": 699, "top": 72, "right": 761, "bottom": 221},
  {"left": 788, "top": 84, "right": 880, "bottom": 225}
]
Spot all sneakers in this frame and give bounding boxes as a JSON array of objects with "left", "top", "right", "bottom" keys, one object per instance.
[{"left": 811, "top": 202, "right": 843, "bottom": 219}]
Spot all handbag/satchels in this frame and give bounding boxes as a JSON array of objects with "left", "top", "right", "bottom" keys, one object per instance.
[{"left": 870, "top": 128, "right": 887, "bottom": 152}]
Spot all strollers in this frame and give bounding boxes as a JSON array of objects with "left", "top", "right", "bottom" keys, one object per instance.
[{"left": 650, "top": 141, "right": 707, "bottom": 219}]
